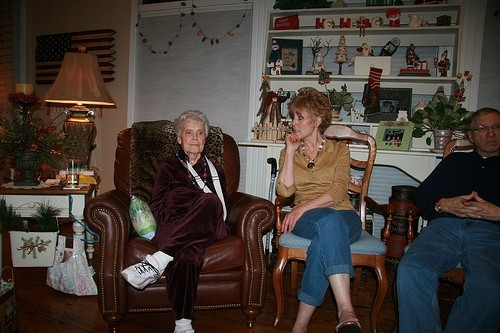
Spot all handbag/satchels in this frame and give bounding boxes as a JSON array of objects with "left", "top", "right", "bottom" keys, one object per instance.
[{"left": 47, "top": 236, "right": 98, "bottom": 295}]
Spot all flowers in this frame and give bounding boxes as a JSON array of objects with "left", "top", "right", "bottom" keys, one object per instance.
[{"left": 7, "top": 92, "right": 41, "bottom": 118}]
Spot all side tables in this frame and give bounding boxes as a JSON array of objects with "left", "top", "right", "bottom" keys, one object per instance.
[{"left": 0, "top": 178, "right": 101, "bottom": 260}]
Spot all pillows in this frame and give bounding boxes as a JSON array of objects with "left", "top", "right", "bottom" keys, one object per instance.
[{"left": 129, "top": 195, "right": 157, "bottom": 240}]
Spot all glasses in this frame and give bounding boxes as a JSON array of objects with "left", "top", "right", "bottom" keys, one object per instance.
[{"left": 468, "top": 124, "right": 500, "bottom": 134}]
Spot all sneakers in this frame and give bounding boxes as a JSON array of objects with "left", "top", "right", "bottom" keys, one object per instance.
[{"left": 120, "top": 253, "right": 164, "bottom": 289}]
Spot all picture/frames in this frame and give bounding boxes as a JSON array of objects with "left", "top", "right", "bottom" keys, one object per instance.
[
  {"left": 375, "top": 121, "right": 414, "bottom": 151},
  {"left": 363, "top": 87, "right": 412, "bottom": 123},
  {"left": 272, "top": 39, "right": 303, "bottom": 75}
]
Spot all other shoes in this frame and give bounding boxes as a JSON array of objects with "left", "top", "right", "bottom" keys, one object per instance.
[{"left": 336, "top": 320, "right": 361, "bottom": 333}]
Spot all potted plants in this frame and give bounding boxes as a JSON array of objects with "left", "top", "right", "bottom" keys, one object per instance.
[
  {"left": 0, "top": 113, "right": 64, "bottom": 186},
  {"left": 327, "top": 89, "right": 354, "bottom": 118},
  {"left": 410, "top": 93, "right": 475, "bottom": 149},
  {"left": 0, "top": 196, "right": 60, "bottom": 267}
]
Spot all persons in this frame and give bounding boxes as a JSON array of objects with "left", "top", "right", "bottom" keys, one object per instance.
[
  {"left": 435, "top": 50, "right": 450, "bottom": 76},
  {"left": 268, "top": 39, "right": 281, "bottom": 72},
  {"left": 406, "top": 43, "right": 415, "bottom": 68},
  {"left": 275, "top": 86, "right": 361, "bottom": 333},
  {"left": 380, "top": 99, "right": 398, "bottom": 113},
  {"left": 397, "top": 107, "right": 500, "bottom": 333},
  {"left": 121, "top": 110, "right": 228, "bottom": 333},
  {"left": 384, "top": 130, "right": 403, "bottom": 146}
]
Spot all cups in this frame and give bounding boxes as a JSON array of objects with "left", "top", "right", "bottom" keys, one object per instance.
[{"left": 65, "top": 157, "right": 82, "bottom": 187}]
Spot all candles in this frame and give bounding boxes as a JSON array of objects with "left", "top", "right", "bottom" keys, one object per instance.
[{"left": 16, "top": 83, "right": 33, "bottom": 95}]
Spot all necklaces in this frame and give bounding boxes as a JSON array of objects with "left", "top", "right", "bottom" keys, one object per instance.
[
  {"left": 301, "top": 135, "right": 326, "bottom": 168},
  {"left": 176, "top": 150, "right": 208, "bottom": 189}
]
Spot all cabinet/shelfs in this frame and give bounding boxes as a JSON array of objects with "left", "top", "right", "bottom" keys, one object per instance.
[{"left": 266, "top": 3, "right": 464, "bottom": 149}]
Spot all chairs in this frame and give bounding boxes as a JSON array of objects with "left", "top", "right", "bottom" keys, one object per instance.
[
  {"left": 402, "top": 139, "right": 473, "bottom": 288},
  {"left": 83, "top": 120, "right": 276, "bottom": 333},
  {"left": 273, "top": 125, "right": 394, "bottom": 333}
]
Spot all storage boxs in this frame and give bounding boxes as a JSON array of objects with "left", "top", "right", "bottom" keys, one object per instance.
[{"left": 354, "top": 56, "right": 392, "bottom": 75}]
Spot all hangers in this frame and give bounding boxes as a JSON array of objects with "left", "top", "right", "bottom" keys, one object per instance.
[{"left": 58, "top": 194, "right": 100, "bottom": 243}]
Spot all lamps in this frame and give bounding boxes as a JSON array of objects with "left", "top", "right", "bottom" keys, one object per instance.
[{"left": 43, "top": 46, "right": 115, "bottom": 149}]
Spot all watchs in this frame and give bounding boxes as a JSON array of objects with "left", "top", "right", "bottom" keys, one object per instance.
[{"left": 435, "top": 201, "right": 441, "bottom": 214}]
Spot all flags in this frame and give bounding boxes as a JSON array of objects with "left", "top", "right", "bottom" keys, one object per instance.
[{"left": 34, "top": 30, "right": 116, "bottom": 84}]
